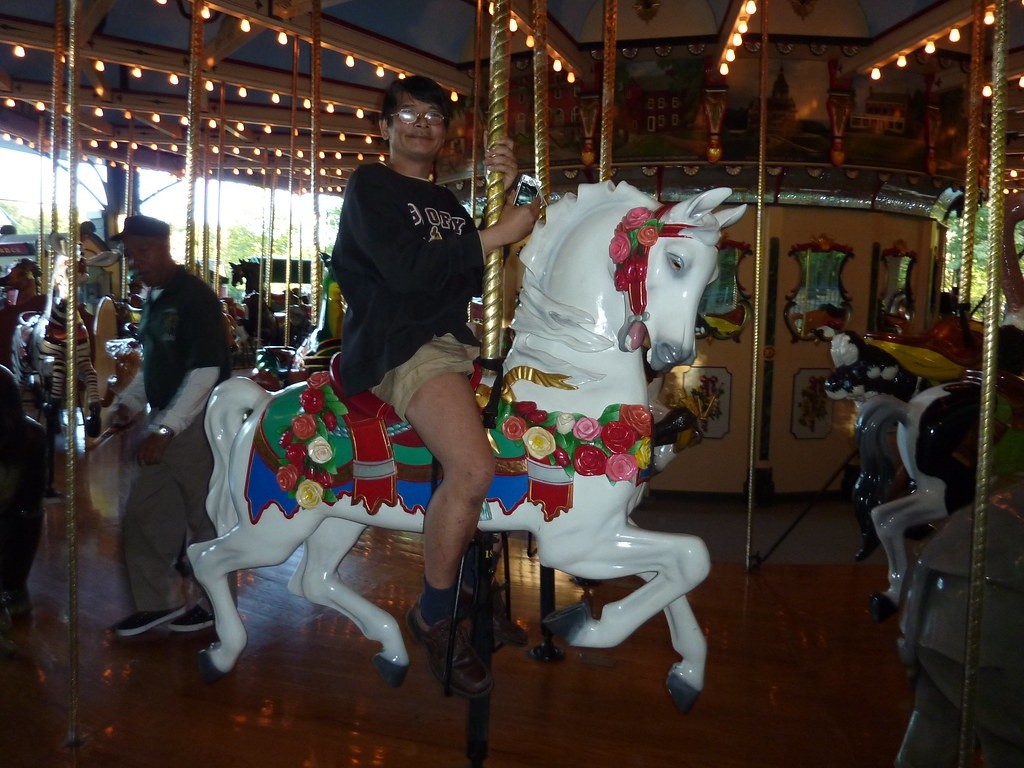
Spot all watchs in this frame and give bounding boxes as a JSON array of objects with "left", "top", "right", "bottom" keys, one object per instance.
[{"left": 158, "top": 426, "right": 172, "bottom": 438}]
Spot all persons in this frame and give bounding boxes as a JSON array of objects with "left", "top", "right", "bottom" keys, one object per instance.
[
  {"left": 107, "top": 215, "right": 239, "bottom": 637},
  {"left": 328, "top": 74, "right": 542, "bottom": 699}
]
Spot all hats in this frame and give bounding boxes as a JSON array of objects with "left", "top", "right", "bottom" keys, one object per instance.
[{"left": 111, "top": 216, "right": 171, "bottom": 243}]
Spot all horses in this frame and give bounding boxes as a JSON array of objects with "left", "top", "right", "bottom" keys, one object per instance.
[
  {"left": 1, "top": 254, "right": 311, "bottom": 438},
  {"left": 185, "top": 178, "right": 748, "bottom": 718},
  {"left": 849, "top": 379, "right": 1024, "bottom": 624}
]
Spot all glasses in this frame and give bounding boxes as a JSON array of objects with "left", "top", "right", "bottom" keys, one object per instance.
[{"left": 391, "top": 109, "right": 445, "bottom": 125}]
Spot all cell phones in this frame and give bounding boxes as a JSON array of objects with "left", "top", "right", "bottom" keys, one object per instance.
[{"left": 513, "top": 174, "right": 543, "bottom": 208}]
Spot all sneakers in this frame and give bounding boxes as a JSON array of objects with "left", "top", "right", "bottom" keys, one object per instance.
[
  {"left": 406, "top": 595, "right": 493, "bottom": 698},
  {"left": 459, "top": 575, "right": 529, "bottom": 646},
  {"left": 116, "top": 604, "right": 186, "bottom": 636},
  {"left": 169, "top": 604, "right": 214, "bottom": 631}
]
